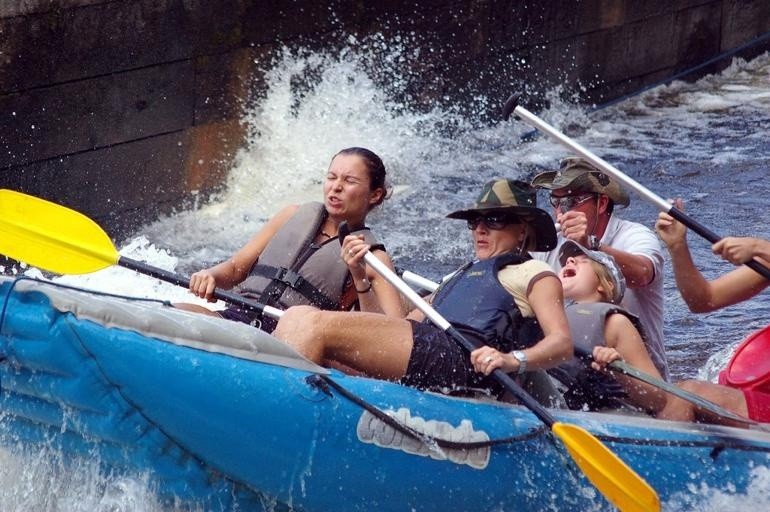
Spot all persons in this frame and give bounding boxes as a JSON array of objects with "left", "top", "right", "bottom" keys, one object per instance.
[
  {"left": 273, "top": 177, "right": 574, "bottom": 399},
  {"left": 163, "top": 144, "right": 394, "bottom": 334},
  {"left": 532, "top": 156, "right": 670, "bottom": 383},
  {"left": 651, "top": 197, "right": 770, "bottom": 431},
  {"left": 494, "top": 239, "right": 671, "bottom": 418}
]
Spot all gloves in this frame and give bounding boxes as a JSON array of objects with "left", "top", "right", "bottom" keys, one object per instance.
[{"left": 567, "top": 370, "right": 627, "bottom": 411}]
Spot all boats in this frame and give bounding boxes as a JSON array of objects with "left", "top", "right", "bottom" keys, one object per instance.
[{"left": 0, "top": 273, "right": 770, "bottom": 512}]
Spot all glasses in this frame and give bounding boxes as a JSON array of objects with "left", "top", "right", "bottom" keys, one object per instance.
[
  {"left": 467, "top": 211, "right": 522, "bottom": 230},
  {"left": 549, "top": 194, "right": 595, "bottom": 213}
]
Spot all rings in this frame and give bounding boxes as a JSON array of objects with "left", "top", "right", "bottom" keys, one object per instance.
[
  {"left": 348, "top": 249, "right": 355, "bottom": 256},
  {"left": 485, "top": 357, "right": 492, "bottom": 362}
]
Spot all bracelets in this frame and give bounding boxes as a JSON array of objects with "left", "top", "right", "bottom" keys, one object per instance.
[{"left": 355, "top": 281, "right": 372, "bottom": 294}]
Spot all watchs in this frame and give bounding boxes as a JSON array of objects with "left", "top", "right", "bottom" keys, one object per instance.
[
  {"left": 588, "top": 234, "right": 603, "bottom": 251},
  {"left": 510, "top": 350, "right": 528, "bottom": 375}
]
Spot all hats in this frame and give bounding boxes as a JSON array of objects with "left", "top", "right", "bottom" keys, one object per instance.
[
  {"left": 445, "top": 179, "right": 558, "bottom": 252},
  {"left": 531, "top": 156, "right": 630, "bottom": 205},
  {"left": 558, "top": 239, "right": 626, "bottom": 305}
]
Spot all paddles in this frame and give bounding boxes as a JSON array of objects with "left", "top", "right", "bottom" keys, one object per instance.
[
  {"left": 394, "top": 266, "right": 760, "bottom": 426},
  {"left": 0, "top": 189, "right": 286, "bottom": 321},
  {"left": 335, "top": 226, "right": 661, "bottom": 512}
]
